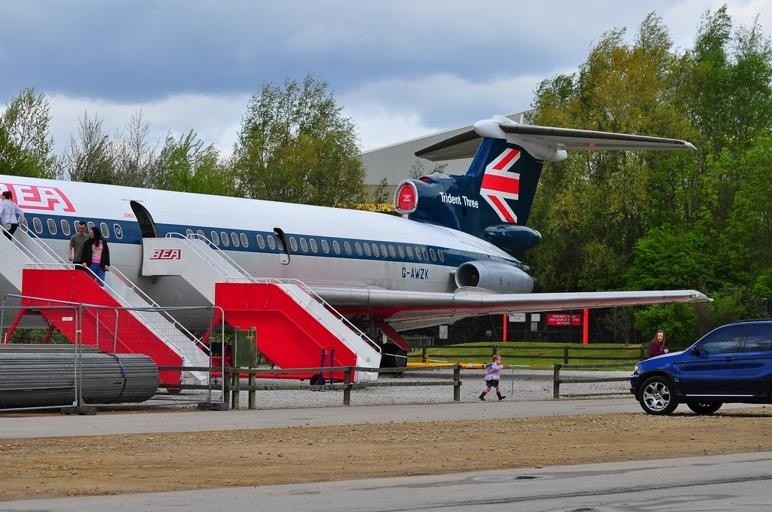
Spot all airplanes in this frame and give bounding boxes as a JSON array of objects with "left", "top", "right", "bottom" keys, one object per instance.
[{"left": 0, "top": 113, "right": 712, "bottom": 373}]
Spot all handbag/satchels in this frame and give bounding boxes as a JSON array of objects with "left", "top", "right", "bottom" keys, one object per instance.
[{"left": 2, "top": 223, "right": 11, "bottom": 232}]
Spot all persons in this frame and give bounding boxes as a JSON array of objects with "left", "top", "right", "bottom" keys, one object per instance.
[
  {"left": 0, "top": 190, "right": 25, "bottom": 241},
  {"left": 478, "top": 353, "right": 507, "bottom": 400},
  {"left": 80, "top": 226, "right": 111, "bottom": 287},
  {"left": 648, "top": 328, "right": 669, "bottom": 358},
  {"left": 67, "top": 221, "right": 91, "bottom": 270}
]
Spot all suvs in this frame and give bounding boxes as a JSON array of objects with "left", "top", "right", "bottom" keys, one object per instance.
[{"left": 631, "top": 319, "right": 772, "bottom": 416}]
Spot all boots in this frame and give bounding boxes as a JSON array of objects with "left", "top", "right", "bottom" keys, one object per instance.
[
  {"left": 478, "top": 392, "right": 486, "bottom": 401},
  {"left": 497, "top": 392, "right": 506, "bottom": 400}
]
